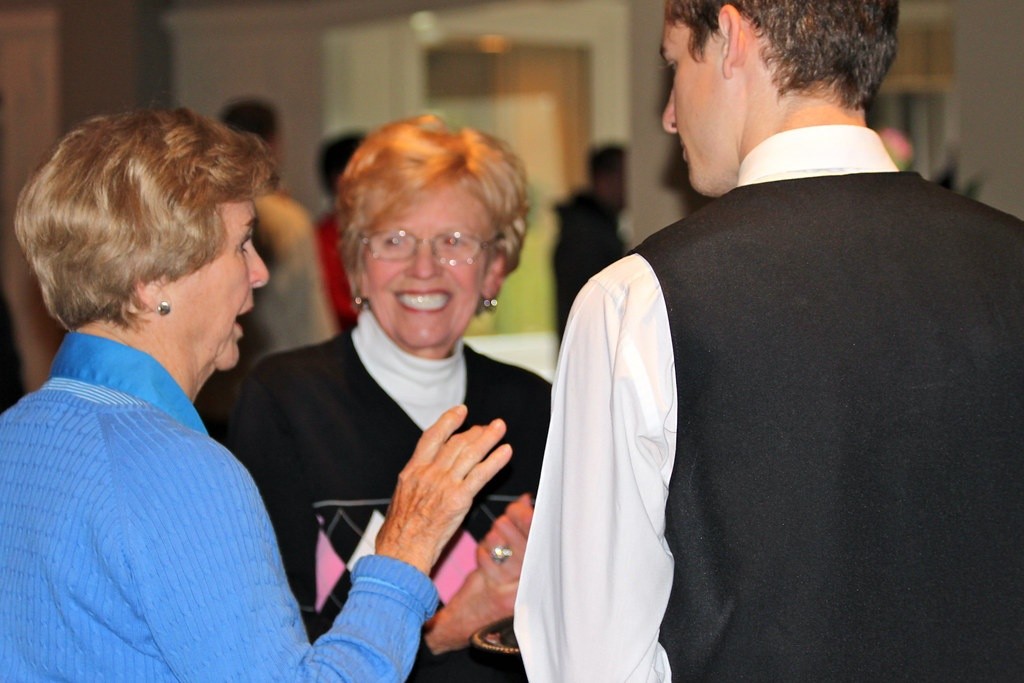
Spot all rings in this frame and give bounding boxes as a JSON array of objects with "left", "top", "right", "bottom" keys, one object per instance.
[{"left": 488, "top": 545, "right": 513, "bottom": 564}]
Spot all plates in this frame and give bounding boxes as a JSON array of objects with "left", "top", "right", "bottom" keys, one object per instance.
[{"left": 471, "top": 613, "right": 522, "bottom": 658}]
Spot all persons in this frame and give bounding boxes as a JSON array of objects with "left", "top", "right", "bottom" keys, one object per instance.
[
  {"left": 216, "top": 94, "right": 336, "bottom": 388},
  {"left": 309, "top": 128, "right": 375, "bottom": 329},
  {"left": 231, "top": 114, "right": 552, "bottom": 683},
  {"left": 517, "top": 0, "right": 1023, "bottom": 683},
  {"left": 0, "top": 105, "right": 517, "bottom": 683},
  {"left": 548, "top": 145, "right": 630, "bottom": 353}
]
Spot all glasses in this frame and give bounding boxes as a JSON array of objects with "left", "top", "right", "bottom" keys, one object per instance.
[{"left": 357, "top": 228, "right": 506, "bottom": 266}]
